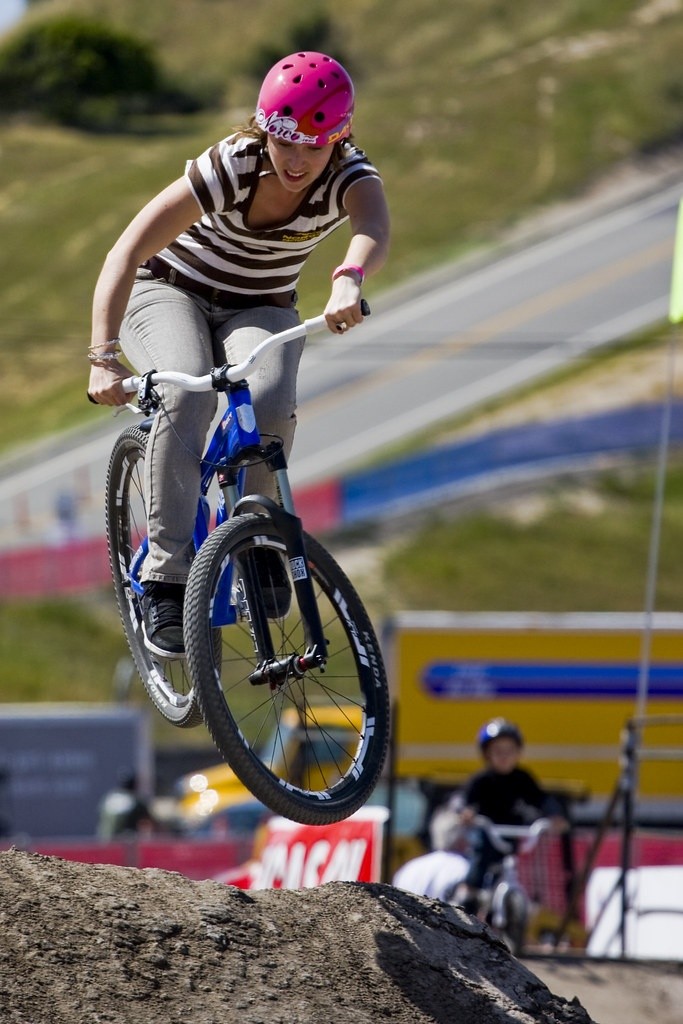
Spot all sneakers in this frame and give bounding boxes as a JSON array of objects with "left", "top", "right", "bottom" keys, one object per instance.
[
  {"left": 235, "top": 546, "right": 292, "bottom": 622},
  {"left": 134, "top": 580, "right": 187, "bottom": 659}
]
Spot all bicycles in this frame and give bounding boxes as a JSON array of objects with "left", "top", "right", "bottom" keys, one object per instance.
[
  {"left": 91, "top": 299, "right": 400, "bottom": 821},
  {"left": 456, "top": 808, "right": 573, "bottom": 963}
]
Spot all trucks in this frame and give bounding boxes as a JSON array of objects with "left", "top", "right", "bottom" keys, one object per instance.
[
  {"left": 171, "top": 610, "right": 683, "bottom": 835},
  {"left": 0, "top": 703, "right": 157, "bottom": 840}
]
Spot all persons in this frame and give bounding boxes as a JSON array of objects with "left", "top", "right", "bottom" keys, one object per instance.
[
  {"left": 454, "top": 717, "right": 565, "bottom": 913},
  {"left": 87, "top": 52, "right": 391, "bottom": 659},
  {"left": 95, "top": 766, "right": 160, "bottom": 842}
]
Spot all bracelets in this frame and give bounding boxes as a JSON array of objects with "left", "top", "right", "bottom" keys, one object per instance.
[
  {"left": 88, "top": 350, "right": 122, "bottom": 360},
  {"left": 87, "top": 338, "right": 121, "bottom": 348},
  {"left": 332, "top": 264, "right": 365, "bottom": 286}
]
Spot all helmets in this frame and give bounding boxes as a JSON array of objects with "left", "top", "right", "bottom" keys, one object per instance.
[
  {"left": 255, "top": 51, "right": 356, "bottom": 147},
  {"left": 479, "top": 720, "right": 523, "bottom": 755}
]
